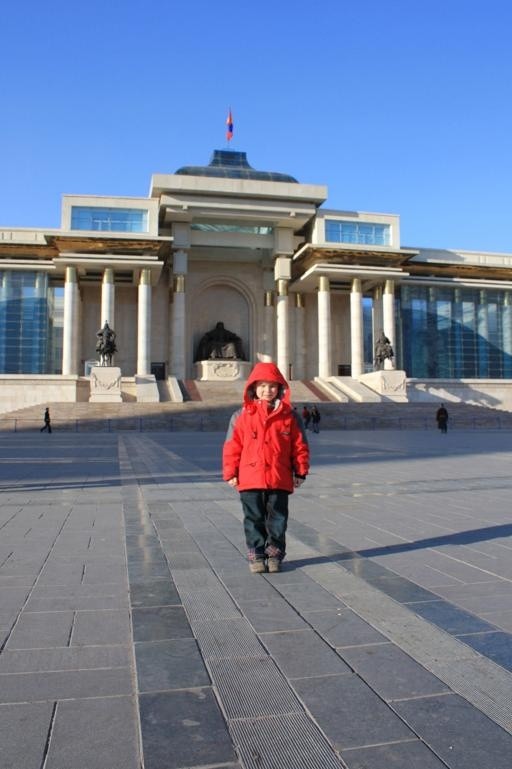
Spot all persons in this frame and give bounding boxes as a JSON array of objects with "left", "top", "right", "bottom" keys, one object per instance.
[
  {"left": 301, "top": 407, "right": 310, "bottom": 429},
  {"left": 436, "top": 403, "right": 448, "bottom": 433},
  {"left": 310, "top": 404, "right": 321, "bottom": 434},
  {"left": 373, "top": 332, "right": 390, "bottom": 356},
  {"left": 94, "top": 323, "right": 116, "bottom": 347},
  {"left": 193, "top": 321, "right": 247, "bottom": 361},
  {"left": 221, "top": 363, "right": 309, "bottom": 575},
  {"left": 40, "top": 408, "right": 53, "bottom": 434}
]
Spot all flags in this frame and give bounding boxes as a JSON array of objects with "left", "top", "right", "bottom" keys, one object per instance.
[{"left": 225, "top": 109, "right": 234, "bottom": 141}]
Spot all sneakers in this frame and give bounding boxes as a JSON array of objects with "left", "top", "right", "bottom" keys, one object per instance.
[
  {"left": 267, "top": 555, "right": 282, "bottom": 573},
  {"left": 247, "top": 558, "right": 267, "bottom": 574}
]
